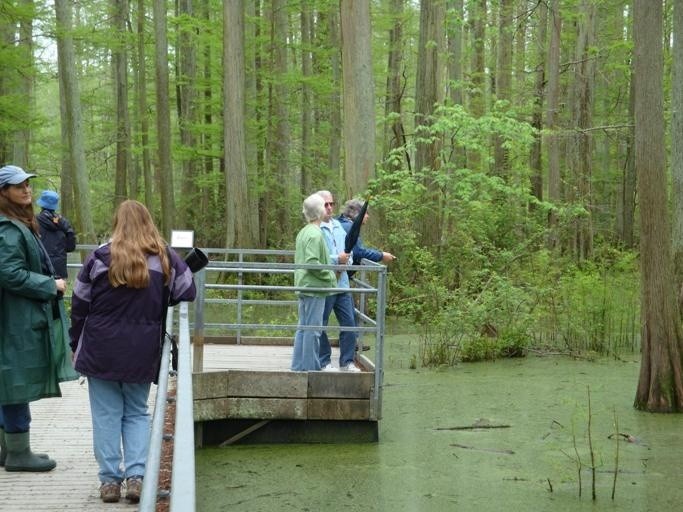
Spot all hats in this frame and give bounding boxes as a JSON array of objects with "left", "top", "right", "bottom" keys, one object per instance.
[
  {"left": 0, "top": 165, "right": 38, "bottom": 189},
  {"left": 36, "top": 190, "right": 59, "bottom": 211}
]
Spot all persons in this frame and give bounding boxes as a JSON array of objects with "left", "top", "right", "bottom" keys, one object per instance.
[
  {"left": 68, "top": 199, "right": 196, "bottom": 503},
  {"left": 0, "top": 163, "right": 66, "bottom": 473},
  {"left": 335, "top": 196, "right": 397, "bottom": 354},
  {"left": 314, "top": 188, "right": 360, "bottom": 373},
  {"left": 292, "top": 193, "right": 335, "bottom": 373},
  {"left": 32, "top": 190, "right": 76, "bottom": 278}
]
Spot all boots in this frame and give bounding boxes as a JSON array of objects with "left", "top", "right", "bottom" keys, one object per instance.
[{"left": 0, "top": 429, "right": 56, "bottom": 472}]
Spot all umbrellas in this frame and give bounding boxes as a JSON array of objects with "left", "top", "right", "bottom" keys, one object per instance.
[{"left": 336, "top": 197, "right": 371, "bottom": 276}]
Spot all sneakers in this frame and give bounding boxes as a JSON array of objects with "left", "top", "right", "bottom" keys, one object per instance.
[
  {"left": 340, "top": 363, "right": 362, "bottom": 373},
  {"left": 126, "top": 477, "right": 142, "bottom": 501},
  {"left": 322, "top": 364, "right": 338, "bottom": 371},
  {"left": 100, "top": 481, "right": 122, "bottom": 502}
]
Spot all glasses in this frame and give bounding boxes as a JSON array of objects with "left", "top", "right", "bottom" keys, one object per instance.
[{"left": 325, "top": 201, "right": 333, "bottom": 208}]
